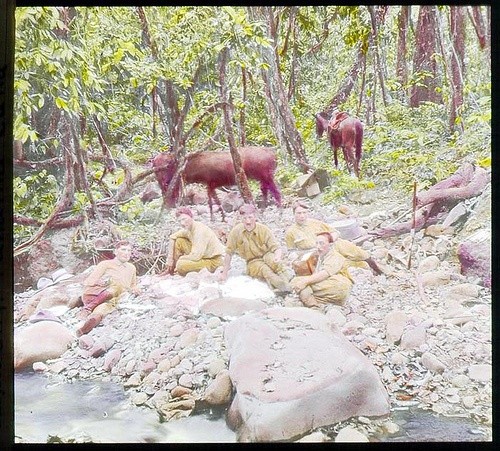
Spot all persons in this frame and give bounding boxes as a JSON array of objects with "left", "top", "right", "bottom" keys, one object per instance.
[
  {"left": 216, "top": 204, "right": 291, "bottom": 291},
  {"left": 156, "top": 360, "right": 230, "bottom": 423},
  {"left": 75, "top": 239, "right": 136, "bottom": 337},
  {"left": 293, "top": 232, "right": 354, "bottom": 312},
  {"left": 286, "top": 200, "right": 372, "bottom": 268},
  {"left": 165, "top": 208, "right": 226, "bottom": 276}
]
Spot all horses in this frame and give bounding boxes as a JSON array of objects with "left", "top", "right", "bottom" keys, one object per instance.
[
  {"left": 312, "top": 110, "right": 366, "bottom": 180},
  {"left": 151, "top": 144, "right": 286, "bottom": 217}
]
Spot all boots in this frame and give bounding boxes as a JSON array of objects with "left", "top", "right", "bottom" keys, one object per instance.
[
  {"left": 76, "top": 313, "right": 101, "bottom": 336},
  {"left": 158, "top": 260, "right": 175, "bottom": 277},
  {"left": 271, "top": 275, "right": 290, "bottom": 297},
  {"left": 84, "top": 290, "right": 113, "bottom": 311},
  {"left": 304, "top": 296, "right": 327, "bottom": 312},
  {"left": 365, "top": 257, "right": 392, "bottom": 276}
]
